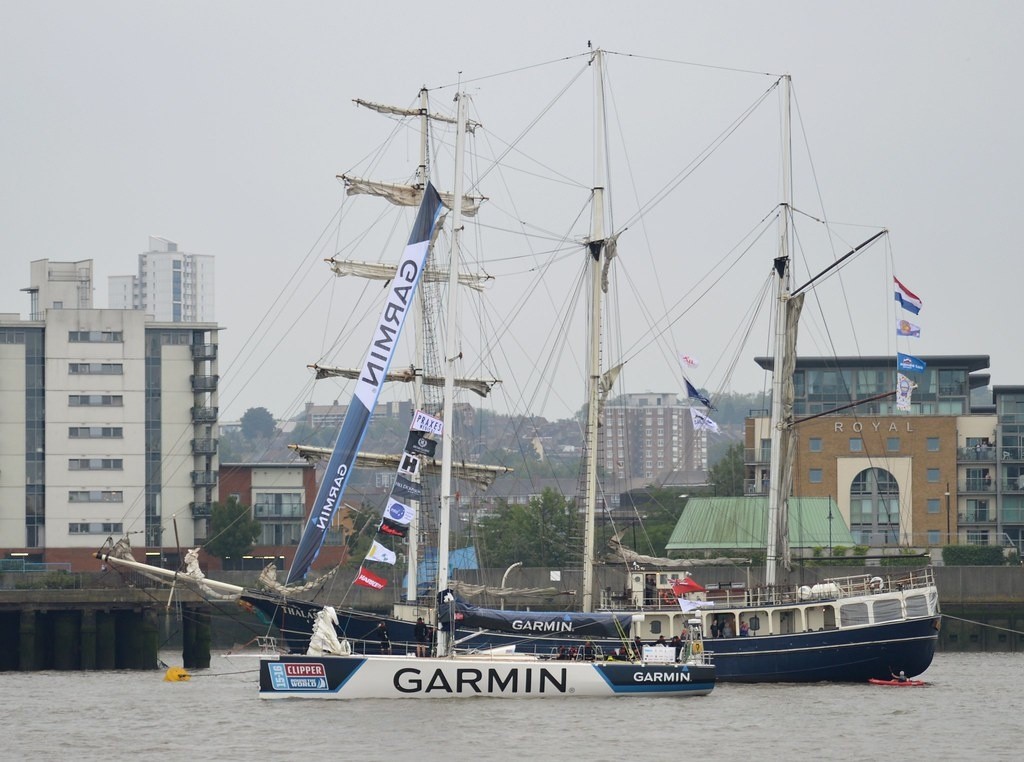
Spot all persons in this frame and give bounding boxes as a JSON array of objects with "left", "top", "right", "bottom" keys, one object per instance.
[
  {"left": 646, "top": 574, "right": 656, "bottom": 605},
  {"left": 377, "top": 621, "right": 392, "bottom": 655},
  {"left": 414, "top": 617, "right": 429, "bottom": 657},
  {"left": 891, "top": 670, "right": 907, "bottom": 682},
  {"left": 557, "top": 628, "right": 690, "bottom": 663},
  {"left": 709, "top": 619, "right": 749, "bottom": 639},
  {"left": 975, "top": 441, "right": 991, "bottom": 491}
]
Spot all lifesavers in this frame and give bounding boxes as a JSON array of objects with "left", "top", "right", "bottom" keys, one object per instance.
[
  {"left": 692, "top": 642, "right": 702, "bottom": 654},
  {"left": 664, "top": 590, "right": 677, "bottom": 604}
]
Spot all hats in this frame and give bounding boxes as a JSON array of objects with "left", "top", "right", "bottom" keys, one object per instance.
[{"left": 660, "top": 635, "right": 665, "bottom": 638}]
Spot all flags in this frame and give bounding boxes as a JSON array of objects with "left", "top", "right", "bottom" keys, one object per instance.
[
  {"left": 896, "top": 318, "right": 920, "bottom": 338},
  {"left": 391, "top": 475, "right": 422, "bottom": 501},
  {"left": 896, "top": 373, "right": 918, "bottom": 411},
  {"left": 365, "top": 540, "right": 396, "bottom": 566},
  {"left": 893, "top": 275, "right": 922, "bottom": 316},
  {"left": 410, "top": 409, "right": 444, "bottom": 436},
  {"left": 898, "top": 352, "right": 927, "bottom": 373},
  {"left": 404, "top": 431, "right": 438, "bottom": 457},
  {"left": 683, "top": 376, "right": 720, "bottom": 413},
  {"left": 397, "top": 452, "right": 421, "bottom": 476},
  {"left": 689, "top": 406, "right": 723, "bottom": 435},
  {"left": 377, "top": 519, "right": 409, "bottom": 538},
  {"left": 352, "top": 566, "right": 387, "bottom": 591},
  {"left": 678, "top": 598, "right": 715, "bottom": 613},
  {"left": 677, "top": 351, "right": 698, "bottom": 368},
  {"left": 383, "top": 496, "right": 416, "bottom": 525},
  {"left": 667, "top": 577, "right": 709, "bottom": 597}
]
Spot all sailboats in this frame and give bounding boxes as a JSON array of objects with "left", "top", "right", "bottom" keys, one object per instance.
[{"left": 93, "top": 53, "right": 941, "bottom": 680}]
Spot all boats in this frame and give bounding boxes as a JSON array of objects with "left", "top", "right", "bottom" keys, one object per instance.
[{"left": 255, "top": 658, "right": 717, "bottom": 696}]
